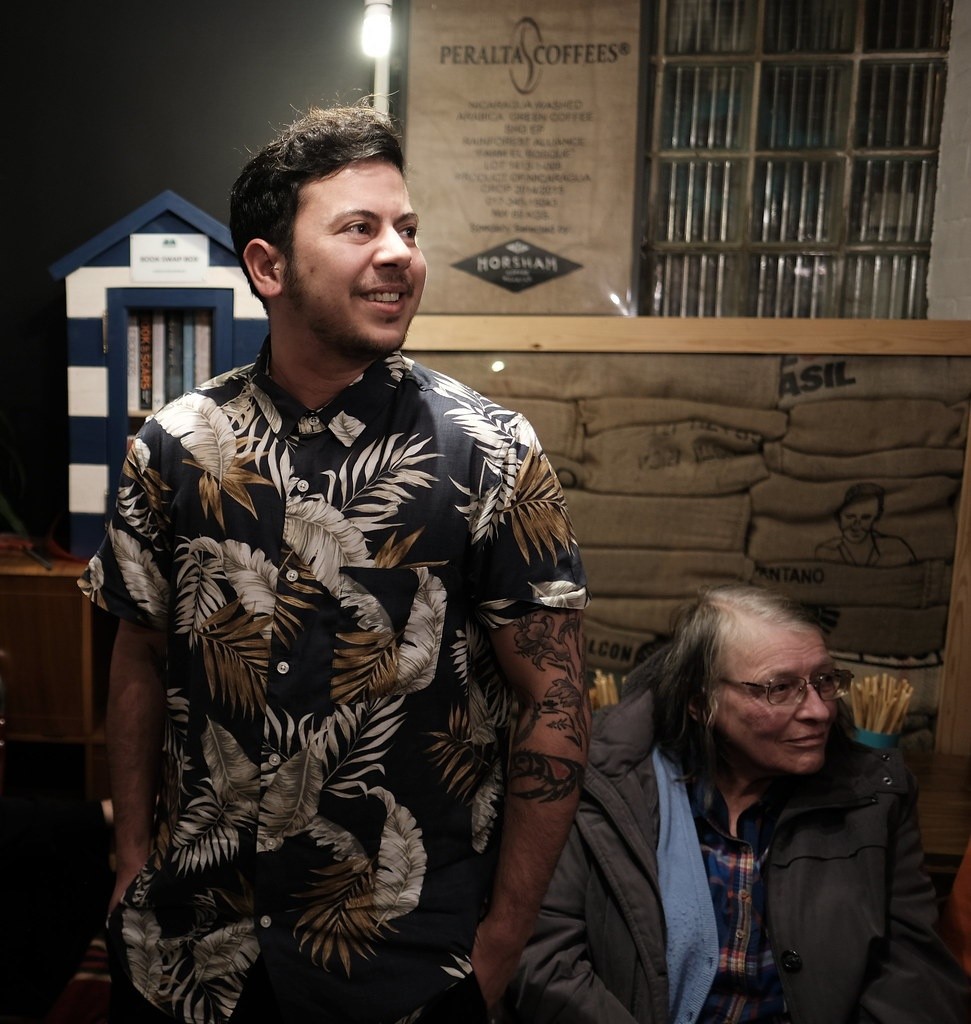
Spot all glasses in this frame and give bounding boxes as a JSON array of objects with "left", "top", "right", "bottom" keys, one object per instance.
[{"left": 717, "top": 669, "right": 855, "bottom": 707}]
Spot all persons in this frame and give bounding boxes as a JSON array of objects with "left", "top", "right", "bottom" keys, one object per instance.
[
  {"left": 498, "top": 583, "right": 971, "bottom": 1024},
  {"left": 77, "top": 105, "right": 592, "bottom": 1024}
]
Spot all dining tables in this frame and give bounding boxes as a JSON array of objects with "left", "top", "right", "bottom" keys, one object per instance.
[{"left": 916, "top": 749, "right": 971, "bottom": 876}]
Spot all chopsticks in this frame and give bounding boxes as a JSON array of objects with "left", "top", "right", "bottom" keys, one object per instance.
[{"left": 850, "top": 673, "right": 914, "bottom": 734}]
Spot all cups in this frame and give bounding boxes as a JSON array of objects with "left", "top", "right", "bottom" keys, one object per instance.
[{"left": 852, "top": 726, "right": 900, "bottom": 749}]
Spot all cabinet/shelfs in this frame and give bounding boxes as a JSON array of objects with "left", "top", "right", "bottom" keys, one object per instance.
[{"left": 1, "top": 553, "right": 108, "bottom": 806}]
